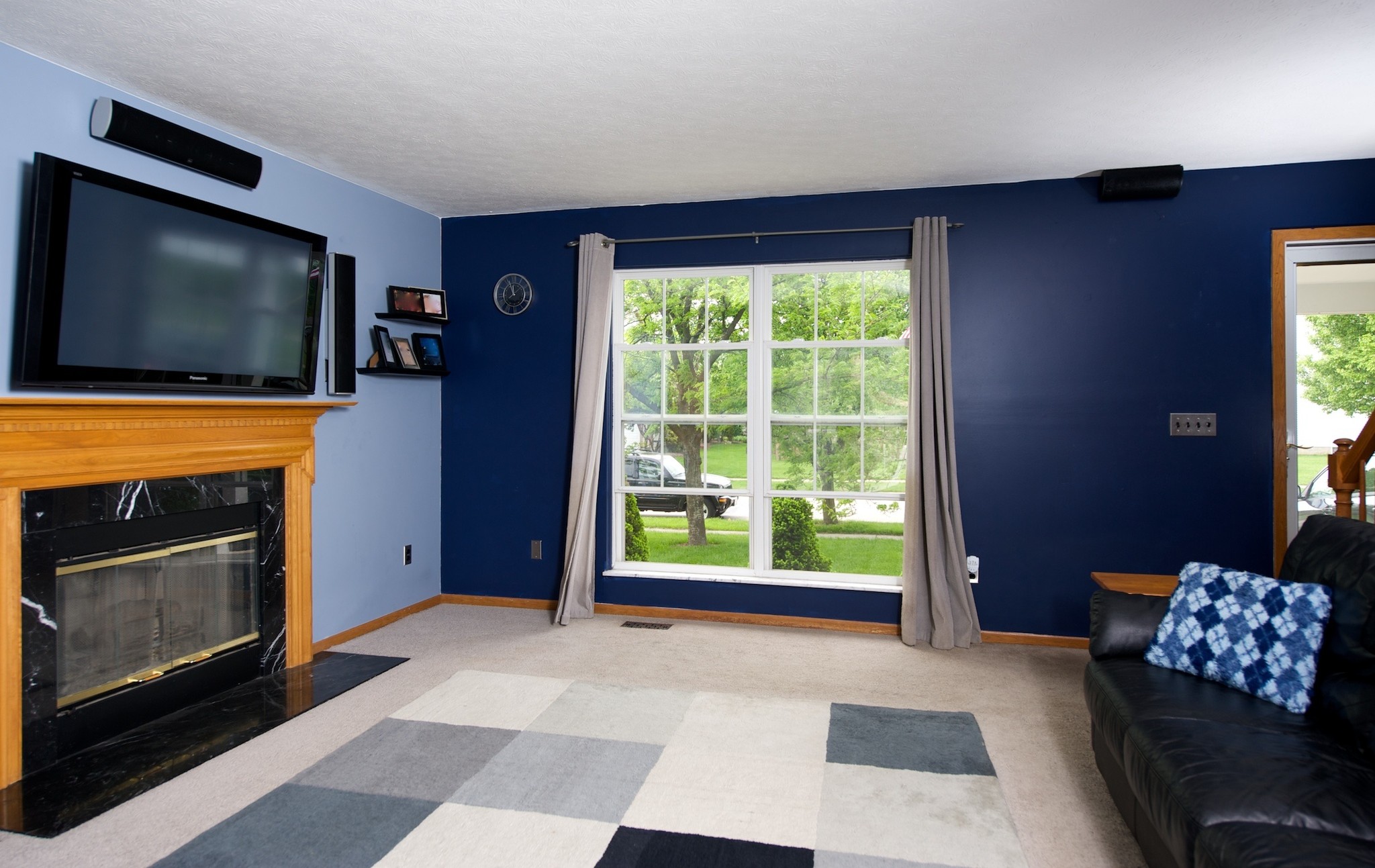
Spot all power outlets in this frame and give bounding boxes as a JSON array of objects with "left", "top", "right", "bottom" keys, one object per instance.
[
  {"left": 403, "top": 545, "right": 412, "bottom": 565},
  {"left": 967, "top": 556, "right": 979, "bottom": 583}
]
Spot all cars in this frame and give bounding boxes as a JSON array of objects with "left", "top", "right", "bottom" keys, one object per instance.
[{"left": 1298, "top": 453, "right": 1375, "bottom": 531}]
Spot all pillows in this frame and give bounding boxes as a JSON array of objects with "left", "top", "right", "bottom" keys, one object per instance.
[{"left": 1145, "top": 562, "right": 1331, "bottom": 714}]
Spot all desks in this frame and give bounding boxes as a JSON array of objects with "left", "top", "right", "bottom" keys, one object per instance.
[{"left": 1091, "top": 572, "right": 1179, "bottom": 596}]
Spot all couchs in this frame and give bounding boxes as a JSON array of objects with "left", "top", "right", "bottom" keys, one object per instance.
[{"left": 1082, "top": 514, "right": 1375, "bottom": 868}]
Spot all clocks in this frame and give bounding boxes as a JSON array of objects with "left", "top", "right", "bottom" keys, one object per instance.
[{"left": 493, "top": 274, "right": 532, "bottom": 315}]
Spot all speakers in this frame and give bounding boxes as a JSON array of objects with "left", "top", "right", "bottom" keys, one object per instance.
[
  {"left": 88, "top": 97, "right": 263, "bottom": 188},
  {"left": 325, "top": 252, "right": 356, "bottom": 395}
]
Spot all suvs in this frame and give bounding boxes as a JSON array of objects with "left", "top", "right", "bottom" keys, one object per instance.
[{"left": 624, "top": 448, "right": 740, "bottom": 519}]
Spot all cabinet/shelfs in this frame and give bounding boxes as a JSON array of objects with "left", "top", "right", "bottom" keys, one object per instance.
[{"left": 357, "top": 313, "right": 452, "bottom": 378}]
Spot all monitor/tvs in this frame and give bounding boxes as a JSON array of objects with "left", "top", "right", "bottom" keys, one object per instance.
[{"left": 10, "top": 152, "right": 328, "bottom": 395}]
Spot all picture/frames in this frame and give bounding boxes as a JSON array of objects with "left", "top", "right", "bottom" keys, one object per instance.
[
  {"left": 369, "top": 325, "right": 447, "bottom": 369},
  {"left": 389, "top": 285, "right": 448, "bottom": 320}
]
